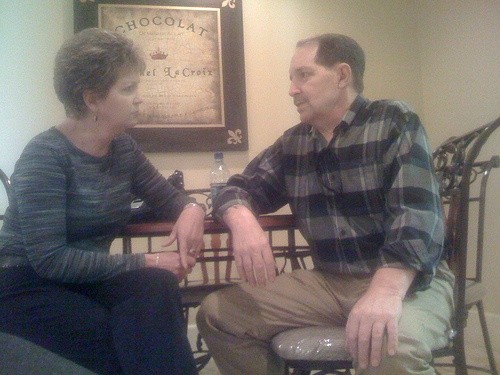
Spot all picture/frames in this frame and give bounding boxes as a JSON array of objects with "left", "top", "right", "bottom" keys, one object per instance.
[{"left": 72, "top": 0, "right": 249, "bottom": 152}]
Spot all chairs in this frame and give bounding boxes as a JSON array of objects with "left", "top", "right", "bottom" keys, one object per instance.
[
  {"left": 269, "top": 117, "right": 500, "bottom": 375},
  {"left": 175, "top": 186, "right": 274, "bottom": 375},
  {"left": 439, "top": 153, "right": 500, "bottom": 375}
]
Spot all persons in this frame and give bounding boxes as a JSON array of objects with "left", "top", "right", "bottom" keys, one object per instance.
[
  {"left": 0, "top": 28, "right": 207, "bottom": 375},
  {"left": 196, "top": 33, "right": 458, "bottom": 375}
]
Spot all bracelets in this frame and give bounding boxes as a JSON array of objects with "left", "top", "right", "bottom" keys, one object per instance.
[
  {"left": 156, "top": 253, "right": 159, "bottom": 267},
  {"left": 190, "top": 202, "right": 198, "bottom": 207}
]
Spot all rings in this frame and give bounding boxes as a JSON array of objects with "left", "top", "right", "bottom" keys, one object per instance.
[{"left": 189, "top": 249, "right": 196, "bottom": 254}]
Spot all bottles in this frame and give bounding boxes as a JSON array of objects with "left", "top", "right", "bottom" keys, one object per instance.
[{"left": 209, "top": 151, "right": 230, "bottom": 209}]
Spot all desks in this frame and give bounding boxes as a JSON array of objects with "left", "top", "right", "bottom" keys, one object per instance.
[{"left": 116, "top": 213, "right": 317, "bottom": 352}]
